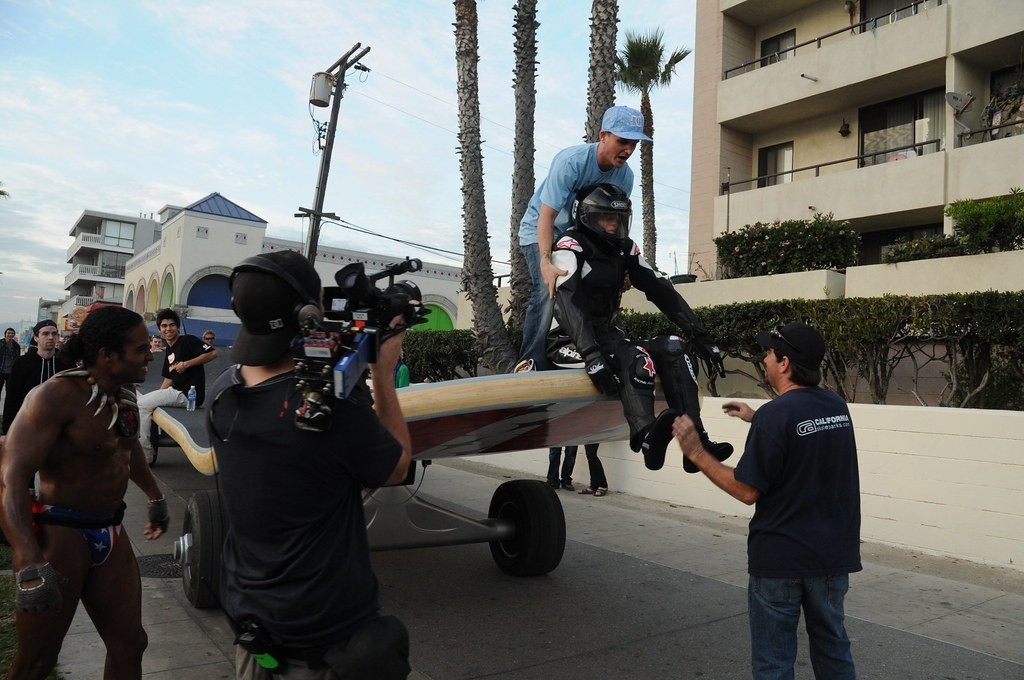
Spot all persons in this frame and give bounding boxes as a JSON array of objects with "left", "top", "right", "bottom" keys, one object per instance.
[
  {"left": 0, "top": 296, "right": 170, "bottom": 680},
  {"left": 546, "top": 443, "right": 609, "bottom": 496},
  {"left": 514, "top": 106, "right": 654, "bottom": 372},
  {"left": 673, "top": 322, "right": 863, "bottom": 680},
  {"left": 205, "top": 251, "right": 412, "bottom": 680},
  {"left": 0, "top": 307, "right": 218, "bottom": 470},
  {"left": 394, "top": 344, "right": 410, "bottom": 388},
  {"left": 548, "top": 183, "right": 733, "bottom": 474}
]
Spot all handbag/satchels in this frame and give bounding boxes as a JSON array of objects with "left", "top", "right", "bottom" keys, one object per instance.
[{"left": 324, "top": 616, "right": 412, "bottom": 680}]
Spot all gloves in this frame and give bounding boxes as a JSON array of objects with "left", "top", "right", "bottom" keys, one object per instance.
[
  {"left": 687, "top": 327, "right": 727, "bottom": 379},
  {"left": 587, "top": 363, "right": 618, "bottom": 397}
]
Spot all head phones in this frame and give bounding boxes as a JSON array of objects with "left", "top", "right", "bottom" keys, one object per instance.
[{"left": 228, "top": 255, "right": 322, "bottom": 335}]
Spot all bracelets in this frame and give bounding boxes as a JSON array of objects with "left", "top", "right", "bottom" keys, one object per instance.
[{"left": 180, "top": 361, "right": 186, "bottom": 371}]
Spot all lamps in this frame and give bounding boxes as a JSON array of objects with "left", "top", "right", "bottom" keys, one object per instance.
[{"left": 838, "top": 118, "right": 851, "bottom": 137}]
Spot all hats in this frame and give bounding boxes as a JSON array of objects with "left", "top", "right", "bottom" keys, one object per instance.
[
  {"left": 757, "top": 324, "right": 826, "bottom": 370},
  {"left": 229, "top": 251, "right": 312, "bottom": 366},
  {"left": 29, "top": 320, "right": 57, "bottom": 346},
  {"left": 601, "top": 105, "right": 653, "bottom": 142}
]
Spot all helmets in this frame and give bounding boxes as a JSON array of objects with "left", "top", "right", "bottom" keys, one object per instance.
[{"left": 571, "top": 182, "right": 633, "bottom": 255}]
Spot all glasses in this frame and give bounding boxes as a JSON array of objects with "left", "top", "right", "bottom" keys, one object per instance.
[
  {"left": 769, "top": 326, "right": 801, "bottom": 353},
  {"left": 153, "top": 334, "right": 161, "bottom": 337},
  {"left": 204, "top": 337, "right": 216, "bottom": 340}
]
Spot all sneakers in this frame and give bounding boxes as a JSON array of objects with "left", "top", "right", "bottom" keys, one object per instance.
[
  {"left": 514, "top": 358, "right": 537, "bottom": 373},
  {"left": 551, "top": 345, "right": 587, "bottom": 369},
  {"left": 641, "top": 408, "right": 679, "bottom": 470},
  {"left": 682, "top": 431, "right": 734, "bottom": 473}
]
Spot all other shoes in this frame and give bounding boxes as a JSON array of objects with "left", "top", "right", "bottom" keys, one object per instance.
[
  {"left": 547, "top": 480, "right": 561, "bottom": 489},
  {"left": 561, "top": 481, "right": 575, "bottom": 490},
  {"left": 144, "top": 447, "right": 154, "bottom": 465}
]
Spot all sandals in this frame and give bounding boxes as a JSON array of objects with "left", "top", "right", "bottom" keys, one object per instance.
[
  {"left": 594, "top": 486, "right": 608, "bottom": 496},
  {"left": 578, "top": 486, "right": 597, "bottom": 494}
]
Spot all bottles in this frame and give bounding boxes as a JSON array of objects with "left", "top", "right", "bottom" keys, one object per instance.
[{"left": 186, "top": 385, "right": 196, "bottom": 412}]
